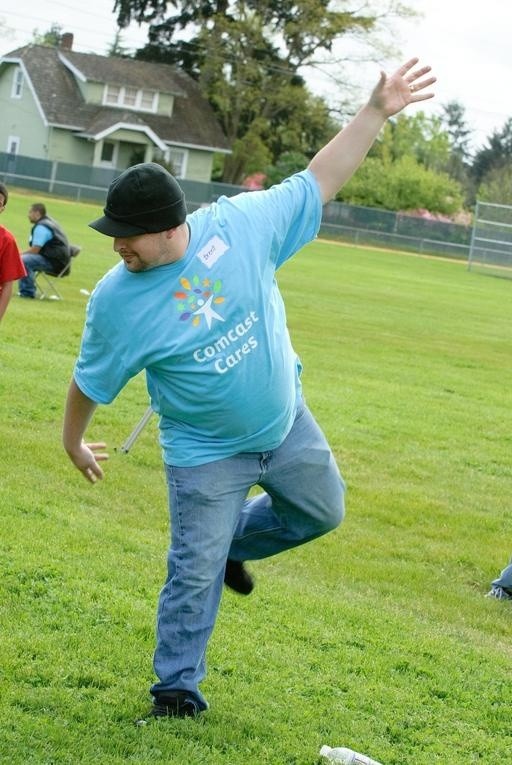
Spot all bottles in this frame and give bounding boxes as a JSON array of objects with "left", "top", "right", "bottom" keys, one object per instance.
[{"left": 318, "top": 743, "right": 383, "bottom": 765}]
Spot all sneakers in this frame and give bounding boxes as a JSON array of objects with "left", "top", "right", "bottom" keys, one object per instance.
[
  {"left": 483, "top": 585, "right": 511, "bottom": 601},
  {"left": 135, "top": 697, "right": 197, "bottom": 726},
  {"left": 225, "top": 558, "right": 252, "bottom": 594}
]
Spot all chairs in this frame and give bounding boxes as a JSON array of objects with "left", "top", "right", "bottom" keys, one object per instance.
[{"left": 28, "top": 246, "right": 81, "bottom": 301}]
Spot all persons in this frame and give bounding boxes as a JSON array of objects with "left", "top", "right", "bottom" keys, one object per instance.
[
  {"left": 0, "top": 181, "right": 28, "bottom": 319},
  {"left": 63, "top": 57, "right": 437, "bottom": 726},
  {"left": 17, "top": 203, "right": 71, "bottom": 299}
]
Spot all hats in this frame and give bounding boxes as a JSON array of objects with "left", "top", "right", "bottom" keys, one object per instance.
[{"left": 88, "top": 162, "right": 187, "bottom": 239}]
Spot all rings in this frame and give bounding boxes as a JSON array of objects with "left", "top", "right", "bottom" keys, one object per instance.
[{"left": 409, "top": 85, "right": 414, "bottom": 93}]
